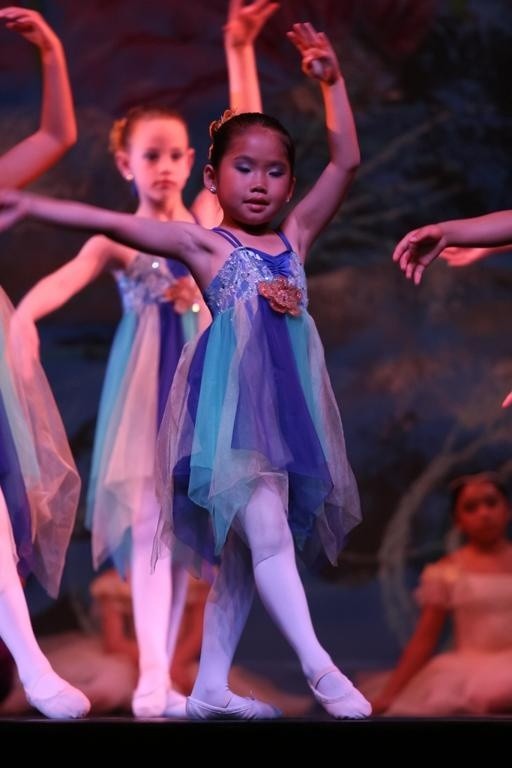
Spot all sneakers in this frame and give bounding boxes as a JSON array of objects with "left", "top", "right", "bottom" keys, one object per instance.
[
  {"left": 305, "top": 661, "right": 373, "bottom": 720},
  {"left": 130, "top": 685, "right": 186, "bottom": 718},
  {"left": 185, "top": 693, "right": 284, "bottom": 721},
  {"left": 19, "top": 670, "right": 92, "bottom": 719}
]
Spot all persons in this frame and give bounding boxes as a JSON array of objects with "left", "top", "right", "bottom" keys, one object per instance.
[
  {"left": 1, "top": 2, "right": 93, "bottom": 722},
  {"left": 438, "top": 245, "right": 512, "bottom": 412},
  {"left": 3, "top": 20, "right": 374, "bottom": 720},
  {"left": 387, "top": 210, "right": 512, "bottom": 289},
  {"left": 8, "top": 0, "right": 284, "bottom": 718},
  {"left": 352, "top": 466, "right": 512, "bottom": 718},
  {"left": 4, "top": 559, "right": 316, "bottom": 719}
]
